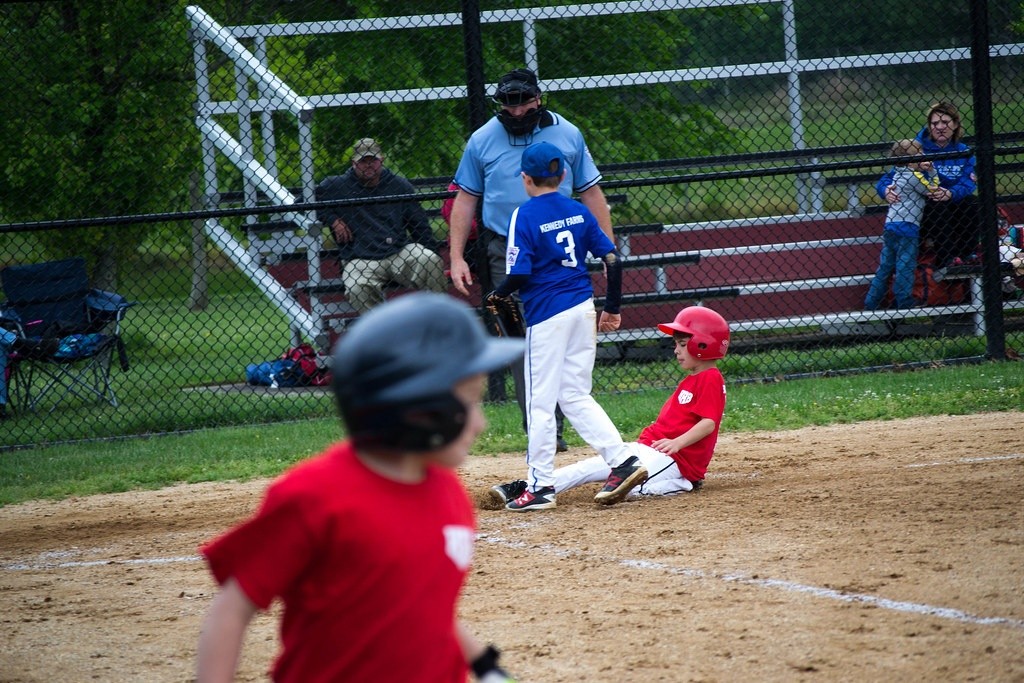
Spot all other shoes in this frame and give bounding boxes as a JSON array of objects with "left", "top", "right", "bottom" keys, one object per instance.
[
  {"left": 900, "top": 298, "right": 927, "bottom": 309},
  {"left": 946, "top": 257, "right": 962, "bottom": 267},
  {"left": 558, "top": 438, "right": 568, "bottom": 452},
  {"left": 29, "top": 338, "right": 60, "bottom": 360},
  {"left": 965, "top": 254, "right": 979, "bottom": 264},
  {"left": 0, "top": 410, "right": 8, "bottom": 418}
]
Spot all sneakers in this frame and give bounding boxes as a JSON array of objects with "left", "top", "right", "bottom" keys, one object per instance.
[
  {"left": 594, "top": 455, "right": 648, "bottom": 505},
  {"left": 489, "top": 480, "right": 528, "bottom": 504},
  {"left": 505, "top": 486, "right": 557, "bottom": 513}
]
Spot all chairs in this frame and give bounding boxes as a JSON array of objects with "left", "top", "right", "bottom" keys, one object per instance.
[{"left": 0, "top": 256, "right": 140, "bottom": 418}]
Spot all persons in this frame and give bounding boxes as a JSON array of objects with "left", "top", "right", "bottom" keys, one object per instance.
[
  {"left": 876, "top": 104, "right": 981, "bottom": 281},
  {"left": 490, "top": 306, "right": 729, "bottom": 501},
  {"left": 194, "top": 291, "right": 511, "bottom": 683},
  {"left": 449, "top": 69, "right": 615, "bottom": 453},
  {"left": 442, "top": 180, "right": 482, "bottom": 275},
  {"left": 0, "top": 326, "right": 60, "bottom": 420},
  {"left": 864, "top": 138, "right": 940, "bottom": 309},
  {"left": 293, "top": 138, "right": 449, "bottom": 314},
  {"left": 484, "top": 140, "right": 647, "bottom": 511}
]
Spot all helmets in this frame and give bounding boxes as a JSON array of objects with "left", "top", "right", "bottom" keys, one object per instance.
[
  {"left": 333, "top": 290, "right": 526, "bottom": 452},
  {"left": 657, "top": 306, "right": 730, "bottom": 361}
]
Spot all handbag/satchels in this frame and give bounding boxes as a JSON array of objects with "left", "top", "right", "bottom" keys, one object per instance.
[
  {"left": 54, "top": 333, "right": 128, "bottom": 373},
  {"left": 245, "top": 344, "right": 332, "bottom": 386}
]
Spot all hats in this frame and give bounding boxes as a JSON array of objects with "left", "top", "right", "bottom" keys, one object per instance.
[
  {"left": 514, "top": 141, "right": 564, "bottom": 177},
  {"left": 353, "top": 137, "right": 382, "bottom": 162},
  {"left": 498, "top": 68, "right": 542, "bottom": 94}
]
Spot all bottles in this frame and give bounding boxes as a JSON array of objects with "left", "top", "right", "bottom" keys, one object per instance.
[
  {"left": 620, "top": 234, "right": 632, "bottom": 258},
  {"left": 657, "top": 267, "right": 666, "bottom": 292}
]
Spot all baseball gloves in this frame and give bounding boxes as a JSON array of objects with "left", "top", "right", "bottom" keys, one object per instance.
[{"left": 481, "top": 291, "right": 526, "bottom": 340}]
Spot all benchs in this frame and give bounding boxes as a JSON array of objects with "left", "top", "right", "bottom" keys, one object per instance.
[{"left": 245, "top": 149, "right": 1024, "bottom": 366}]
